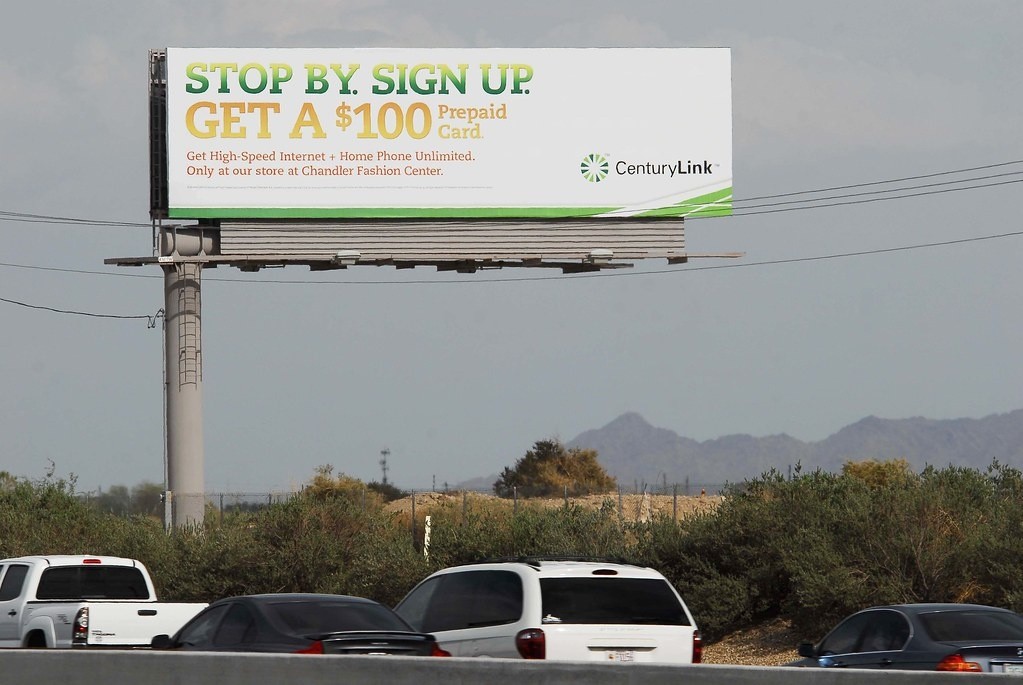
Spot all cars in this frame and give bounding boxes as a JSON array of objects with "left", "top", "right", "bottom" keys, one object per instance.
[
  {"left": 779, "top": 600, "right": 1023, "bottom": 676},
  {"left": 148, "top": 589, "right": 452, "bottom": 658}
]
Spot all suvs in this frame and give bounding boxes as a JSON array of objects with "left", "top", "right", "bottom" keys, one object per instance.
[{"left": 389, "top": 553, "right": 703, "bottom": 664}]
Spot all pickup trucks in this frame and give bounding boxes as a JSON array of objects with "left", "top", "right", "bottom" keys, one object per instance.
[{"left": 0, "top": 554, "right": 208, "bottom": 651}]
